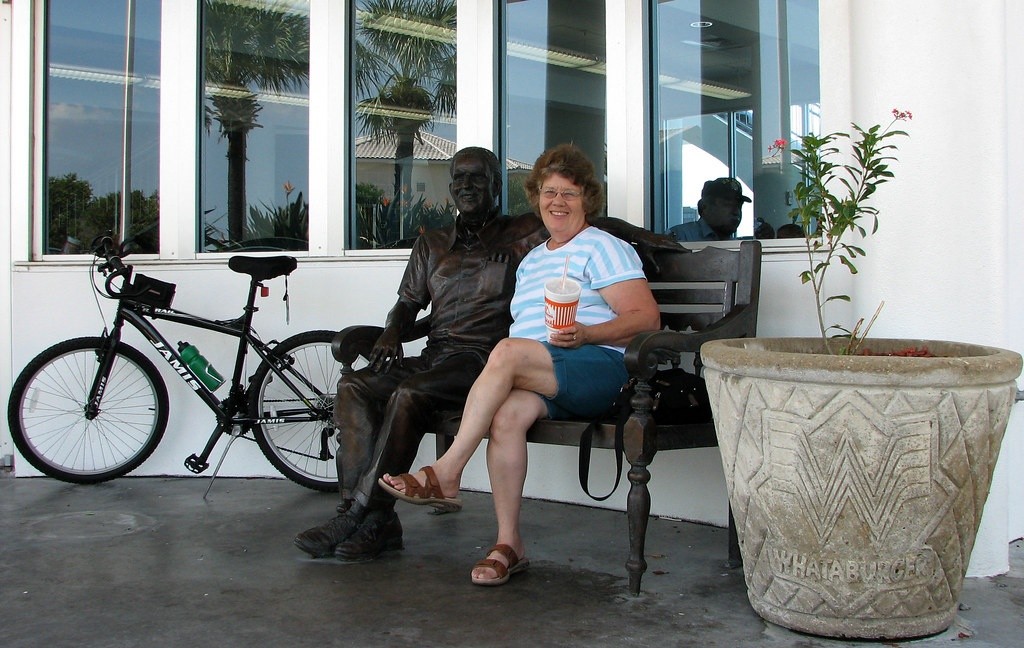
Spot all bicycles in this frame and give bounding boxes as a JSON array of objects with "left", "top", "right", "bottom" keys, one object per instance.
[{"left": 7, "top": 228, "right": 375, "bottom": 499}]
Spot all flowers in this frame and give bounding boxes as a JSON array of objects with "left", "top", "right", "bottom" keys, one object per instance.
[{"left": 766, "top": 107, "right": 912, "bottom": 356}]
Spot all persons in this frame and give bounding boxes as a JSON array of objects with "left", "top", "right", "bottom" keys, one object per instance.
[
  {"left": 378, "top": 145, "right": 660, "bottom": 586},
  {"left": 669, "top": 177, "right": 752, "bottom": 242},
  {"left": 294, "top": 146, "right": 692, "bottom": 563}
]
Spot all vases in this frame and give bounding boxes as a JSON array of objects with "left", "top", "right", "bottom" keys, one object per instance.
[{"left": 700, "top": 336, "right": 1024, "bottom": 642}]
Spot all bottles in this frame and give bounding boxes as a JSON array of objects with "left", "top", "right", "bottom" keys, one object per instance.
[{"left": 177, "top": 341, "right": 227, "bottom": 393}]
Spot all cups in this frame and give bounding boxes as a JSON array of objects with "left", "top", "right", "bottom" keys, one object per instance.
[
  {"left": 544, "top": 277, "right": 582, "bottom": 343},
  {"left": 62, "top": 237, "right": 82, "bottom": 254}
]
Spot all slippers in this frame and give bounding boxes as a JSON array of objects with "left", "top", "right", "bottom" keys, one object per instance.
[
  {"left": 378, "top": 466, "right": 463, "bottom": 513},
  {"left": 470, "top": 544, "right": 529, "bottom": 586}
]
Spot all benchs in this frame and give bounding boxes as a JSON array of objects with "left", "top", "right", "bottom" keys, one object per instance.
[{"left": 327, "top": 239, "right": 762, "bottom": 600}]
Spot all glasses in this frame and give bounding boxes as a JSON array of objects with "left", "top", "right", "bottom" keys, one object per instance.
[{"left": 538, "top": 184, "right": 583, "bottom": 201}]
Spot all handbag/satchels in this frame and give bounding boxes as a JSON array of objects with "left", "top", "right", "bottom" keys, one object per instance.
[{"left": 579, "top": 367, "right": 713, "bottom": 501}]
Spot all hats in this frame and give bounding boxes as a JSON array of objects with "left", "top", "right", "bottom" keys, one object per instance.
[{"left": 702, "top": 177, "right": 752, "bottom": 203}]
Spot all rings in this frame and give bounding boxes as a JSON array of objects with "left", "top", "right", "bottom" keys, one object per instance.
[{"left": 573, "top": 334, "right": 577, "bottom": 342}]
